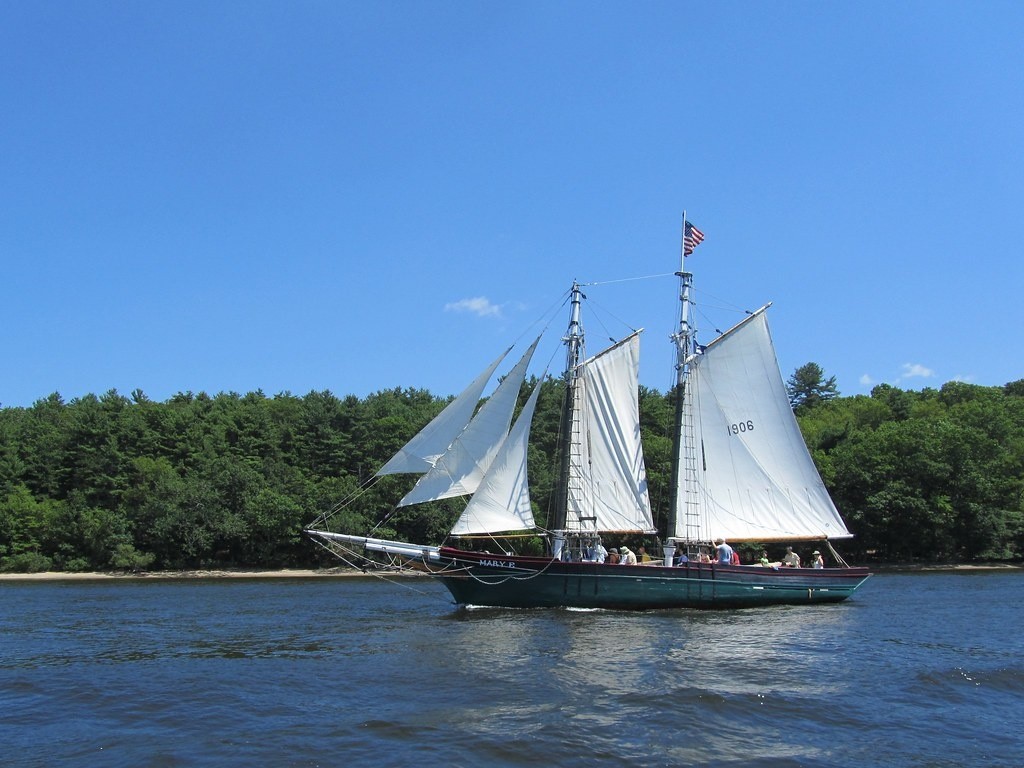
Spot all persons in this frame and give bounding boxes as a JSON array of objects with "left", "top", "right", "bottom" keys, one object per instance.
[
  {"left": 582, "top": 539, "right": 608, "bottom": 563},
  {"left": 620, "top": 546, "right": 637, "bottom": 564},
  {"left": 610, "top": 548, "right": 620, "bottom": 564},
  {"left": 785, "top": 547, "right": 805, "bottom": 568},
  {"left": 732, "top": 547, "right": 740, "bottom": 565},
  {"left": 760, "top": 550, "right": 768, "bottom": 566},
  {"left": 639, "top": 547, "right": 651, "bottom": 562},
  {"left": 700, "top": 551, "right": 719, "bottom": 564},
  {"left": 678, "top": 549, "right": 688, "bottom": 564},
  {"left": 812, "top": 551, "right": 823, "bottom": 569},
  {"left": 711, "top": 537, "right": 733, "bottom": 564}
]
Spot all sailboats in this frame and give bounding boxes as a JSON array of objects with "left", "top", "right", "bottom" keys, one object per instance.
[{"left": 304, "top": 213, "right": 873, "bottom": 604}]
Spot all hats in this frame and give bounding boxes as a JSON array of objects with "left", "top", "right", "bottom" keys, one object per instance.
[
  {"left": 610, "top": 548, "right": 618, "bottom": 553},
  {"left": 812, "top": 550, "right": 821, "bottom": 555},
  {"left": 620, "top": 546, "right": 629, "bottom": 553}
]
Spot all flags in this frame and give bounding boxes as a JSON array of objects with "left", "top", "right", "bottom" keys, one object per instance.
[{"left": 684, "top": 221, "right": 706, "bottom": 257}]
